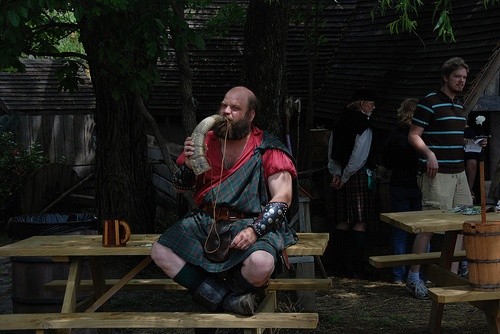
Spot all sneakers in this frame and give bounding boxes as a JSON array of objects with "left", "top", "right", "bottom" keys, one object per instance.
[
  {"left": 405, "top": 279, "right": 431, "bottom": 299},
  {"left": 458, "top": 262, "right": 468, "bottom": 278}
]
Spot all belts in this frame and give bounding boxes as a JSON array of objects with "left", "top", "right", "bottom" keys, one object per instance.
[{"left": 199, "top": 199, "right": 260, "bottom": 221}]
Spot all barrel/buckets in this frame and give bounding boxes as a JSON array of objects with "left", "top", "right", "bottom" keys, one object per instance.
[{"left": 462, "top": 221, "right": 500, "bottom": 292}]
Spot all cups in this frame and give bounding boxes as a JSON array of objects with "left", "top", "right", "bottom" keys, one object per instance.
[{"left": 102, "top": 219, "right": 131, "bottom": 248}]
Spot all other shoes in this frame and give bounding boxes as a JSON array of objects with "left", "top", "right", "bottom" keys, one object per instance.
[
  {"left": 340, "top": 273, "right": 361, "bottom": 284},
  {"left": 194, "top": 272, "right": 267, "bottom": 316}
]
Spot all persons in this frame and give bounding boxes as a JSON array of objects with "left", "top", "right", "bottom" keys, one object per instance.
[
  {"left": 327, "top": 89, "right": 386, "bottom": 280},
  {"left": 406, "top": 57, "right": 487, "bottom": 299},
  {"left": 382, "top": 98, "right": 433, "bottom": 284},
  {"left": 151, "top": 86, "right": 299, "bottom": 334}
]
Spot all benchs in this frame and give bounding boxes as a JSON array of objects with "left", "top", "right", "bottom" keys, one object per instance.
[
  {"left": 45, "top": 278, "right": 332, "bottom": 292},
  {"left": 381, "top": 210, "right": 500, "bottom": 334},
  {"left": 427, "top": 285, "right": 500, "bottom": 303},
  {"left": 0, "top": 313, "right": 318, "bottom": 329}
]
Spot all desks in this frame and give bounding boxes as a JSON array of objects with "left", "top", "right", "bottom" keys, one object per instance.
[
  {"left": 369, "top": 249, "right": 466, "bottom": 266},
  {"left": 0, "top": 233, "right": 330, "bottom": 334}
]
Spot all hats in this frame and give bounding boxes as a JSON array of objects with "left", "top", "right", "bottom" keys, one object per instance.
[{"left": 352, "top": 88, "right": 378, "bottom": 101}]
